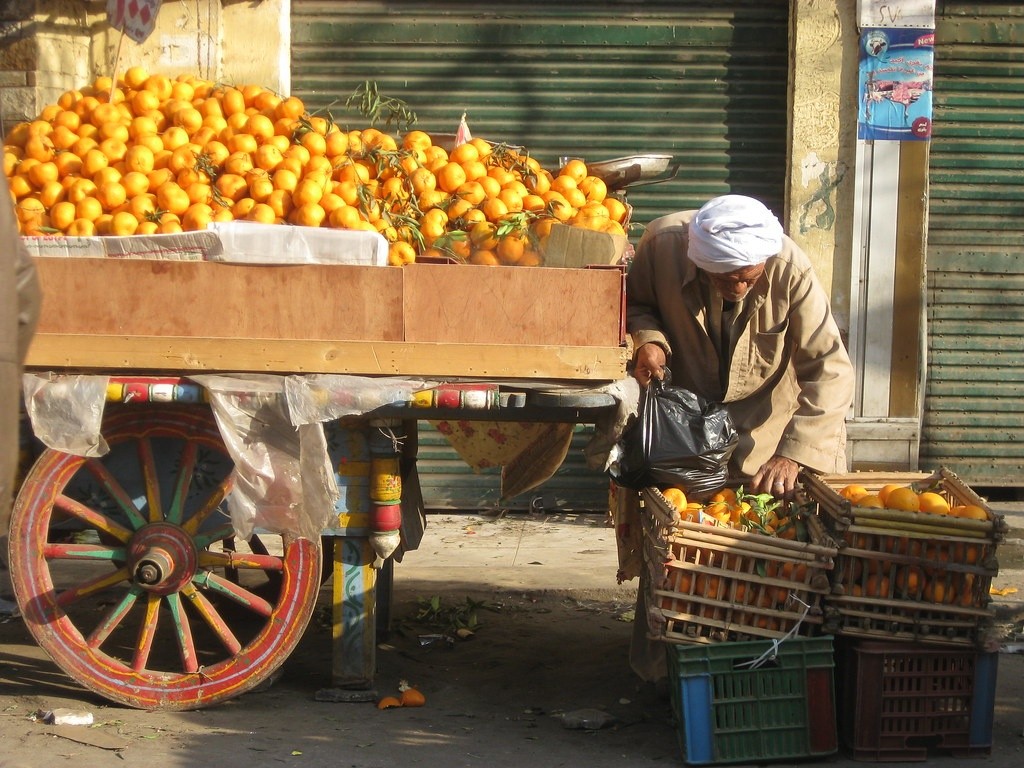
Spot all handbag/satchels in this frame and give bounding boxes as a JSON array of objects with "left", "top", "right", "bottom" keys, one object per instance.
[{"left": 608, "top": 363, "right": 741, "bottom": 495}]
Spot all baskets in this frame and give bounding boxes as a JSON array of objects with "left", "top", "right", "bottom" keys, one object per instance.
[
  {"left": 798, "top": 466, "right": 1010, "bottom": 643},
  {"left": 842, "top": 639, "right": 1000, "bottom": 761},
  {"left": 639, "top": 486, "right": 838, "bottom": 647},
  {"left": 666, "top": 633, "right": 839, "bottom": 764}
]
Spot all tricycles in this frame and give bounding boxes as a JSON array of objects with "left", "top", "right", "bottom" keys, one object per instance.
[{"left": 0, "top": 76, "right": 638, "bottom": 716}]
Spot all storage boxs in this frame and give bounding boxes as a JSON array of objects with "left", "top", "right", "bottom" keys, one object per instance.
[
  {"left": 641, "top": 481, "right": 838, "bottom": 644},
  {"left": 799, "top": 466, "right": 1009, "bottom": 648},
  {"left": 672, "top": 634, "right": 837, "bottom": 768},
  {"left": 845, "top": 641, "right": 999, "bottom": 764}
]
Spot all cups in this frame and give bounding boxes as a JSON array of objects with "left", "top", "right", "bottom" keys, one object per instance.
[{"left": 559, "top": 157, "right": 585, "bottom": 168}]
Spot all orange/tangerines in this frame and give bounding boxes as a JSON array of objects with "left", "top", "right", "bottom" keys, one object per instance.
[{"left": 0, "top": 68, "right": 990, "bottom": 643}]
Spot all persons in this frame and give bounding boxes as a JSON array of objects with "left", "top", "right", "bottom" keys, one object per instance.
[{"left": 622, "top": 193, "right": 856, "bottom": 499}]
